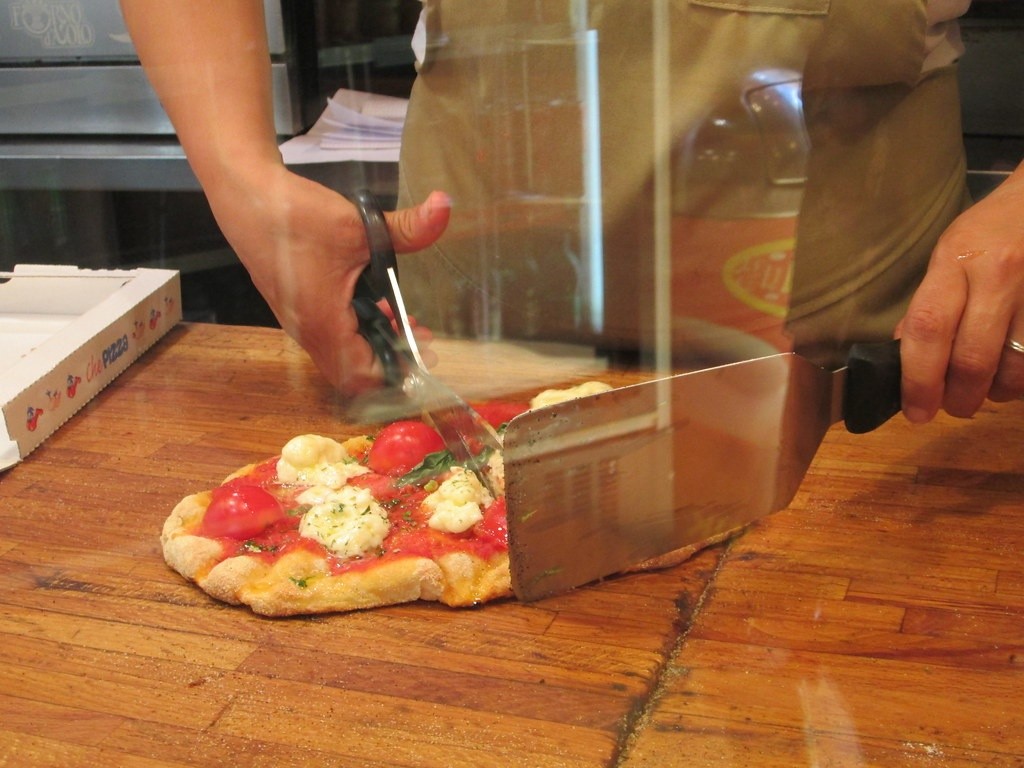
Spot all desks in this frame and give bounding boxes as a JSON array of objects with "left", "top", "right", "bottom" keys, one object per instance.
[
  {"left": 0, "top": 320, "right": 1024, "bottom": 767},
  {"left": 0, "top": 76, "right": 1024, "bottom": 320}
]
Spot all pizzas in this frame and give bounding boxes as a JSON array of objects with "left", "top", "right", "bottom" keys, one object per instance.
[{"left": 160, "top": 381, "right": 740, "bottom": 617}]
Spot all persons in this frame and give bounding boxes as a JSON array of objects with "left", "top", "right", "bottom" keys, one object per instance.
[{"left": 120, "top": 0, "right": 1024, "bottom": 424}]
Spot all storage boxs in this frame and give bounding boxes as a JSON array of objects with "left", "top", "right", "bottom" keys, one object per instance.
[{"left": 0, "top": 264, "right": 183, "bottom": 473}]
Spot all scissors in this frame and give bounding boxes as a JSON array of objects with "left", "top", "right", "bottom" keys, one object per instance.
[{"left": 350, "top": 184, "right": 508, "bottom": 502}]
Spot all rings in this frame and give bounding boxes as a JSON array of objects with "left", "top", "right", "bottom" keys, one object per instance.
[{"left": 1006, "top": 339, "right": 1024, "bottom": 353}]
